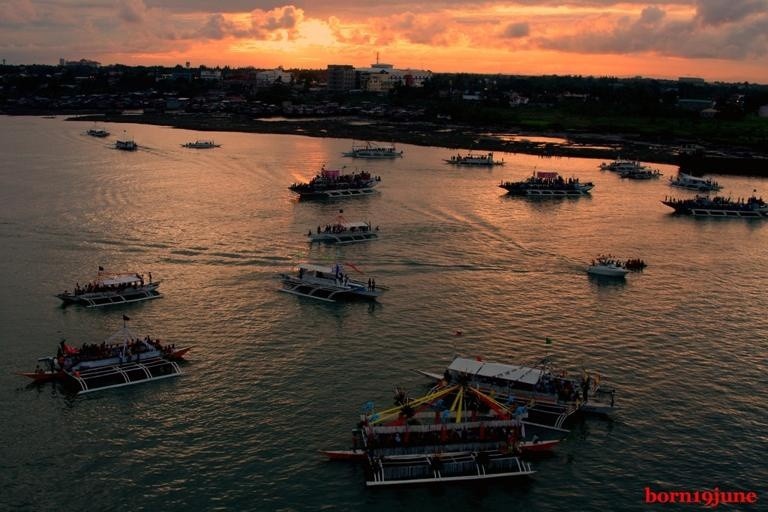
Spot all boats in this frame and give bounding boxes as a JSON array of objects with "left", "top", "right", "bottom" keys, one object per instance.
[
  {"left": 185, "top": 143, "right": 216, "bottom": 148},
  {"left": 288, "top": 164, "right": 381, "bottom": 198},
  {"left": 597, "top": 153, "right": 768, "bottom": 215},
  {"left": 86, "top": 128, "right": 134, "bottom": 151},
  {"left": 497, "top": 169, "right": 595, "bottom": 196},
  {"left": 586, "top": 258, "right": 648, "bottom": 278},
  {"left": 10, "top": 272, "right": 196, "bottom": 397},
  {"left": 341, "top": 142, "right": 403, "bottom": 158},
  {"left": 442, "top": 156, "right": 504, "bottom": 165},
  {"left": 274, "top": 208, "right": 391, "bottom": 305},
  {"left": 317, "top": 358, "right": 622, "bottom": 491}
]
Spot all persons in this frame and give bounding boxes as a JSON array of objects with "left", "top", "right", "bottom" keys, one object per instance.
[
  {"left": 63, "top": 269, "right": 153, "bottom": 296},
  {"left": 591, "top": 252, "right": 644, "bottom": 268},
  {"left": 317, "top": 223, "right": 346, "bottom": 233},
  {"left": 444, "top": 370, "right": 603, "bottom": 405},
  {"left": 351, "top": 434, "right": 358, "bottom": 453},
  {"left": 368, "top": 277, "right": 372, "bottom": 291},
  {"left": 316, "top": 169, "right": 381, "bottom": 182},
  {"left": 299, "top": 267, "right": 304, "bottom": 278},
  {"left": 36, "top": 336, "right": 177, "bottom": 375},
  {"left": 335, "top": 265, "right": 350, "bottom": 286},
  {"left": 372, "top": 278, "right": 375, "bottom": 291}
]
